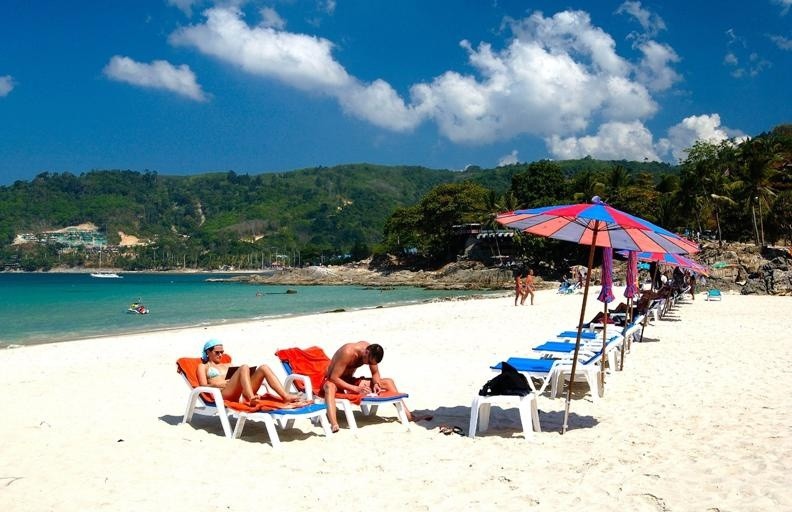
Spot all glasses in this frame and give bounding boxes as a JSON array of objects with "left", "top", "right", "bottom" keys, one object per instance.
[{"left": 214, "top": 351, "right": 224, "bottom": 355}]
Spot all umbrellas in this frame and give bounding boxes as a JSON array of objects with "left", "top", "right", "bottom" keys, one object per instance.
[
  {"left": 613, "top": 250, "right": 710, "bottom": 344},
  {"left": 655, "top": 259, "right": 710, "bottom": 318},
  {"left": 627, "top": 250, "right": 640, "bottom": 353},
  {"left": 597, "top": 247, "right": 615, "bottom": 397},
  {"left": 494, "top": 196, "right": 704, "bottom": 435},
  {"left": 620, "top": 251, "right": 634, "bottom": 371}
]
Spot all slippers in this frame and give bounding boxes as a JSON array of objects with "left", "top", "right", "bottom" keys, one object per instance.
[
  {"left": 440, "top": 424, "right": 452, "bottom": 434},
  {"left": 453, "top": 426, "right": 465, "bottom": 435}
]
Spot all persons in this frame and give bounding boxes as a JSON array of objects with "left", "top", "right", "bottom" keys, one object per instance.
[
  {"left": 521, "top": 269, "right": 535, "bottom": 306},
  {"left": 514, "top": 273, "right": 524, "bottom": 307},
  {"left": 197, "top": 337, "right": 302, "bottom": 408},
  {"left": 321, "top": 340, "right": 432, "bottom": 433},
  {"left": 578, "top": 271, "right": 582, "bottom": 288}
]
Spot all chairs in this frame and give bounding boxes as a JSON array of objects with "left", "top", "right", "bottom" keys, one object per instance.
[
  {"left": 707, "top": 288, "right": 722, "bottom": 302},
  {"left": 468, "top": 281, "right": 693, "bottom": 441},
  {"left": 276, "top": 345, "right": 412, "bottom": 442},
  {"left": 176, "top": 353, "right": 335, "bottom": 449}
]
